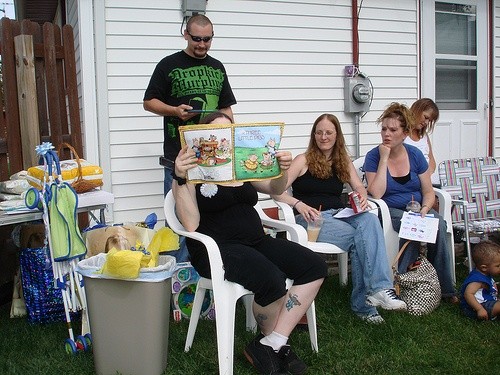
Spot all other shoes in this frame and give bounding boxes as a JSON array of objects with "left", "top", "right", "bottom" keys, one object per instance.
[{"left": 447, "top": 293, "right": 463, "bottom": 308}]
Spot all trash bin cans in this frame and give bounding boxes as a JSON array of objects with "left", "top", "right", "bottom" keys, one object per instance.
[{"left": 76, "top": 252, "right": 175, "bottom": 375}]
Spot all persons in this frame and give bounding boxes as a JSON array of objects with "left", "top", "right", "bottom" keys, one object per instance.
[
  {"left": 170, "top": 114, "right": 326, "bottom": 375},
  {"left": 402, "top": 97, "right": 439, "bottom": 174},
  {"left": 271, "top": 114, "right": 408, "bottom": 324},
  {"left": 363, "top": 103, "right": 458, "bottom": 305},
  {"left": 462, "top": 240, "right": 500, "bottom": 321},
  {"left": 144, "top": 13, "right": 237, "bottom": 265}
]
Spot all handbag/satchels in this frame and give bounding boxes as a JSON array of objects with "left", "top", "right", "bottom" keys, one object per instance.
[
  {"left": 9, "top": 274, "right": 26, "bottom": 318},
  {"left": 394, "top": 240, "right": 441, "bottom": 316},
  {"left": 20, "top": 246, "right": 77, "bottom": 322}
]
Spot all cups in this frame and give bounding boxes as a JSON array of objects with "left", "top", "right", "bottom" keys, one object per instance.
[
  {"left": 307, "top": 211, "right": 323, "bottom": 242},
  {"left": 406, "top": 200, "right": 421, "bottom": 213}
]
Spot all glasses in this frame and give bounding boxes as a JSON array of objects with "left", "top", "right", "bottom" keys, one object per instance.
[
  {"left": 186, "top": 29, "right": 213, "bottom": 42},
  {"left": 314, "top": 131, "right": 336, "bottom": 136}
]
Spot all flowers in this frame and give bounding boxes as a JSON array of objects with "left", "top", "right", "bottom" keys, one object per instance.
[{"left": 35, "top": 142, "right": 55, "bottom": 156}]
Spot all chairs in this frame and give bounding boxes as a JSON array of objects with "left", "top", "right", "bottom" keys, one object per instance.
[
  {"left": 271, "top": 185, "right": 378, "bottom": 287},
  {"left": 163, "top": 187, "right": 318, "bottom": 375},
  {"left": 438, "top": 157, "right": 500, "bottom": 272},
  {"left": 352, "top": 156, "right": 457, "bottom": 285}
]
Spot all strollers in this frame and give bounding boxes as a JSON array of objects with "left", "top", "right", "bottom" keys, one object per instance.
[{"left": 24, "top": 151, "right": 93, "bottom": 357}]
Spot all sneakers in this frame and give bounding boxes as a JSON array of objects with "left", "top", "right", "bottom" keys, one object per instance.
[
  {"left": 244, "top": 332, "right": 306, "bottom": 375},
  {"left": 358, "top": 314, "right": 385, "bottom": 325},
  {"left": 365, "top": 290, "right": 407, "bottom": 312}
]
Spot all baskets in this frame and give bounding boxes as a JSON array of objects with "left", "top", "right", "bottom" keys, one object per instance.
[{"left": 58, "top": 143, "right": 104, "bottom": 194}]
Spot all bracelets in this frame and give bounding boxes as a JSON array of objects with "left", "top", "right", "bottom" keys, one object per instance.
[
  {"left": 170, "top": 172, "right": 186, "bottom": 186},
  {"left": 294, "top": 200, "right": 301, "bottom": 206}
]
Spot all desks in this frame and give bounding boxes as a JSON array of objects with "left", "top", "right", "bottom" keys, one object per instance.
[{"left": 0, "top": 191, "right": 114, "bottom": 225}]
